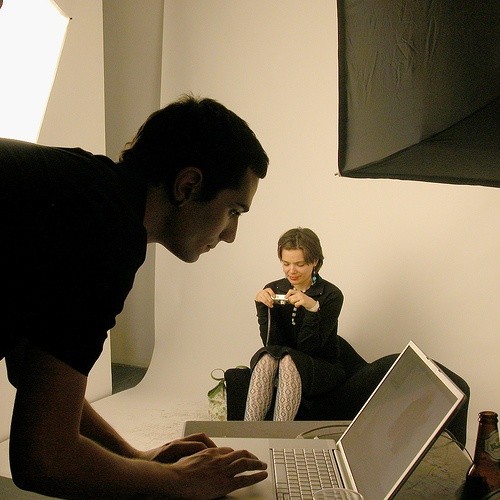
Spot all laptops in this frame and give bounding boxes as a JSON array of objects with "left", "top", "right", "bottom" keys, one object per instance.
[{"left": 208, "top": 339, "right": 468, "bottom": 500}]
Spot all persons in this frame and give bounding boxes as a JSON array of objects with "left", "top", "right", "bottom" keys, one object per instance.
[
  {"left": 0, "top": 95, "right": 270, "bottom": 498},
  {"left": 245, "top": 226, "right": 344, "bottom": 421}
]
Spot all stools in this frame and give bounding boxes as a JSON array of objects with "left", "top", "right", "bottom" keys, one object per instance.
[{"left": 342, "top": 347, "right": 469, "bottom": 452}]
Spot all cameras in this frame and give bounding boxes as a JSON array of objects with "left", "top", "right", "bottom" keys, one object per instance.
[{"left": 270, "top": 294, "right": 291, "bottom": 306}]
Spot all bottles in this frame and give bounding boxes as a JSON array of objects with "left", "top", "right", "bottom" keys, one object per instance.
[{"left": 461, "top": 410, "right": 500, "bottom": 500}]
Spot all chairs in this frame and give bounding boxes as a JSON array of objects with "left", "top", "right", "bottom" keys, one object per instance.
[{"left": 224, "top": 332, "right": 373, "bottom": 428}]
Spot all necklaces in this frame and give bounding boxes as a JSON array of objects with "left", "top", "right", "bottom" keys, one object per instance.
[{"left": 291, "top": 282, "right": 314, "bottom": 326}]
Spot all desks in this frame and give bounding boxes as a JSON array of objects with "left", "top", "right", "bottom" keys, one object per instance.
[{"left": 182, "top": 421, "right": 472, "bottom": 500}]
